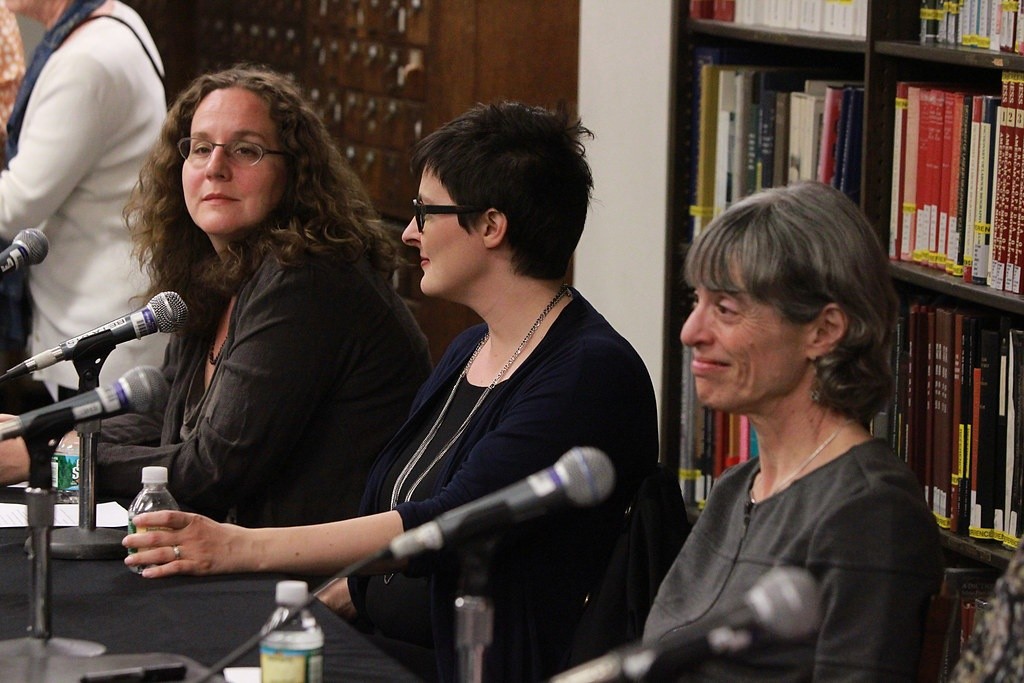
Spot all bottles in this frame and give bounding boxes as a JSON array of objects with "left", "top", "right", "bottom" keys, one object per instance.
[
  {"left": 51, "top": 431, "right": 80, "bottom": 503},
  {"left": 259, "top": 580, "right": 324, "bottom": 683},
  {"left": 127, "top": 466, "right": 179, "bottom": 575}
]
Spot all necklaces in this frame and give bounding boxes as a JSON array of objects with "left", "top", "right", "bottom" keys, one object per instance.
[
  {"left": 208, "top": 334, "right": 227, "bottom": 364},
  {"left": 750, "top": 417, "right": 854, "bottom": 503},
  {"left": 390, "top": 279, "right": 570, "bottom": 511}
]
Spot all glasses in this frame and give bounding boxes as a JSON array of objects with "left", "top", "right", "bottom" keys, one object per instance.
[
  {"left": 176, "top": 137, "right": 292, "bottom": 167},
  {"left": 413, "top": 198, "right": 490, "bottom": 234}
]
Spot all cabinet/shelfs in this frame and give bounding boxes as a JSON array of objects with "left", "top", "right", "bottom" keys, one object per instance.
[{"left": 661, "top": 0, "right": 1024, "bottom": 683}]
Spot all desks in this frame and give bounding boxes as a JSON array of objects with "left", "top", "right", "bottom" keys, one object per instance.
[{"left": 2, "top": 481, "right": 413, "bottom": 682}]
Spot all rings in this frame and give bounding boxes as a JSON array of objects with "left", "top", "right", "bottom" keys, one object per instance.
[{"left": 173, "top": 546, "right": 180, "bottom": 559}]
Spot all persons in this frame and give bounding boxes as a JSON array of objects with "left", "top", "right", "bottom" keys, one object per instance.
[
  {"left": 949, "top": 541, "right": 1024, "bottom": 683},
  {"left": 0, "top": 0, "right": 173, "bottom": 420},
  {"left": 122, "top": 99, "right": 659, "bottom": 683},
  {"left": 546, "top": 180, "right": 949, "bottom": 683},
  {"left": 0, "top": 61, "right": 433, "bottom": 527}
]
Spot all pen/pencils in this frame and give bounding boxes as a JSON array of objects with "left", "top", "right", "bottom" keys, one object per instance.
[{"left": 78, "top": 662, "right": 188, "bottom": 683}]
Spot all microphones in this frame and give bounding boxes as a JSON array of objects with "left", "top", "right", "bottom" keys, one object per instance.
[
  {"left": 382, "top": 446, "right": 616, "bottom": 562},
  {"left": 0, "top": 366, "right": 171, "bottom": 444},
  {"left": 0, "top": 228, "right": 49, "bottom": 280},
  {"left": 551, "top": 566, "right": 823, "bottom": 683},
  {"left": 7, "top": 291, "right": 188, "bottom": 378}
]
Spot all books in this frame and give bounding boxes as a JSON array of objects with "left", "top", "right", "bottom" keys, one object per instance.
[{"left": 677, "top": 0, "right": 1024, "bottom": 555}]
